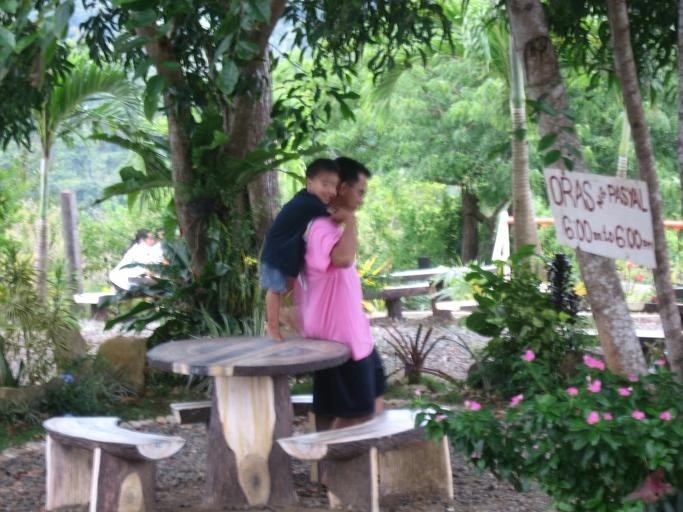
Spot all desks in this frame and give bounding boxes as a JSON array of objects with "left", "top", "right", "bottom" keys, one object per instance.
[
  {"left": 145, "top": 336, "right": 350, "bottom": 511},
  {"left": 389, "top": 265, "right": 498, "bottom": 280}
]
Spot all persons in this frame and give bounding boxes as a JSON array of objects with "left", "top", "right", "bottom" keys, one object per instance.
[
  {"left": 294, "top": 157, "right": 387, "bottom": 496},
  {"left": 106, "top": 230, "right": 155, "bottom": 290},
  {"left": 152, "top": 228, "right": 180, "bottom": 281},
  {"left": 259, "top": 158, "right": 341, "bottom": 343}
]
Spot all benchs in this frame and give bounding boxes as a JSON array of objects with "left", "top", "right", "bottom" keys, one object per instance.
[
  {"left": 275, "top": 410, "right": 455, "bottom": 512},
  {"left": 433, "top": 301, "right": 479, "bottom": 318},
  {"left": 73, "top": 293, "right": 118, "bottom": 321},
  {"left": 380, "top": 281, "right": 430, "bottom": 317},
  {"left": 40, "top": 416, "right": 187, "bottom": 512},
  {"left": 169, "top": 395, "right": 319, "bottom": 484}
]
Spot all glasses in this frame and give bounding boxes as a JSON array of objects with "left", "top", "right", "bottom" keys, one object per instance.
[{"left": 350, "top": 189, "right": 367, "bottom": 196}]
